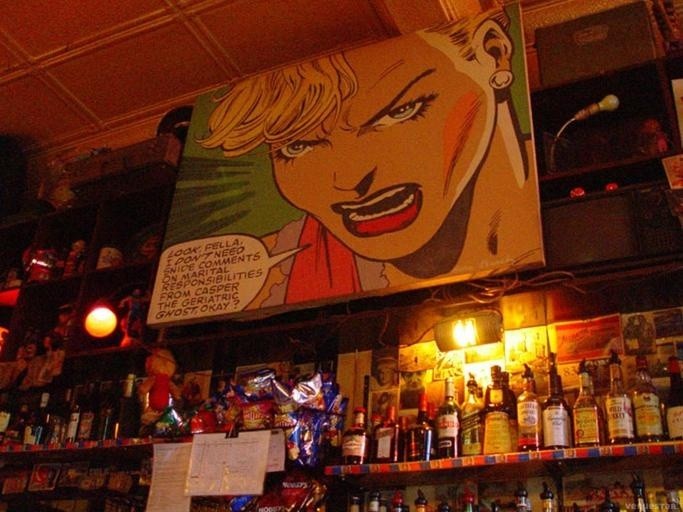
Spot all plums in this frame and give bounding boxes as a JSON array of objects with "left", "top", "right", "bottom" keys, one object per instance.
[
  {"left": 570, "top": 188, "right": 585, "bottom": 198},
  {"left": 605, "top": 183, "right": 618, "bottom": 193}
]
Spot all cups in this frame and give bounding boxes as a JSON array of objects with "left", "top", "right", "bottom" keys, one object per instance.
[
  {"left": 95, "top": 247, "right": 124, "bottom": 269},
  {"left": 43, "top": 187, "right": 76, "bottom": 209}
]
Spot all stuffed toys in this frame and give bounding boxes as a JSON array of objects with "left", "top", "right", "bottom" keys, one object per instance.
[{"left": 137, "top": 347, "right": 181, "bottom": 415}]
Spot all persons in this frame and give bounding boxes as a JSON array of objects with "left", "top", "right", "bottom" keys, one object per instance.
[
  {"left": 0, "top": 331, "right": 65, "bottom": 388},
  {"left": 193, "top": 7, "right": 543, "bottom": 306}
]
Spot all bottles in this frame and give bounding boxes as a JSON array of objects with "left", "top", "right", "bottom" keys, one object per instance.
[
  {"left": 1, "top": 368, "right": 138, "bottom": 443},
  {"left": 338, "top": 348, "right": 683, "bottom": 511}
]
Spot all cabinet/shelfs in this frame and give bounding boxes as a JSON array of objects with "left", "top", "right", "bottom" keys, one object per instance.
[
  {"left": 0, "top": 178, "right": 171, "bottom": 363},
  {"left": 529, "top": 48, "right": 683, "bottom": 276}
]
